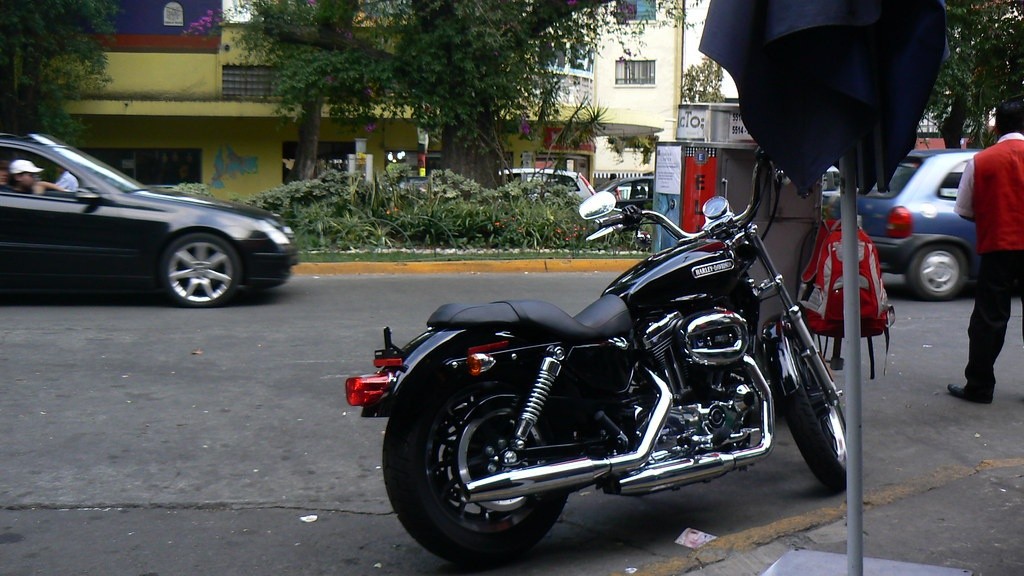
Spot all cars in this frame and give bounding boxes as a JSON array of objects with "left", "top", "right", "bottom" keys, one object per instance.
[
  {"left": 0, "top": 130, "right": 300, "bottom": 308},
  {"left": 822, "top": 148, "right": 985, "bottom": 304},
  {"left": 591, "top": 175, "right": 655, "bottom": 218},
  {"left": 497, "top": 166, "right": 594, "bottom": 205}
]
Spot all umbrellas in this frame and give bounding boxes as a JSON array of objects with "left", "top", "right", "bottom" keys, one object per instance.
[{"left": 695, "top": 0, "right": 947, "bottom": 576}]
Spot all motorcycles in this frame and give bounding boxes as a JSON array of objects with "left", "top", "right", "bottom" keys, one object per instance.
[{"left": 343, "top": 146, "right": 849, "bottom": 569}]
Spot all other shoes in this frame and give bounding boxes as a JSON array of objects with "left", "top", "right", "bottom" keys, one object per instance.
[{"left": 947, "top": 383, "right": 993, "bottom": 403}]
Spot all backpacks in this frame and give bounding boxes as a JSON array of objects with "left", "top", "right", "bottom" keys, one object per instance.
[{"left": 801, "top": 219, "right": 888, "bottom": 337}]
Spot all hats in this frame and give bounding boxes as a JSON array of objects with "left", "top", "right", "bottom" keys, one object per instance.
[
  {"left": 10, "top": 160, "right": 44, "bottom": 173},
  {"left": 992, "top": 100, "right": 1024, "bottom": 130}
]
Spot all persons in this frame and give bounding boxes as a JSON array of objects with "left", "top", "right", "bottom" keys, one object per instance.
[
  {"left": 946, "top": 100, "right": 1024, "bottom": 404},
  {"left": 610, "top": 174, "right": 621, "bottom": 201},
  {"left": 0, "top": 155, "right": 79, "bottom": 196}
]
